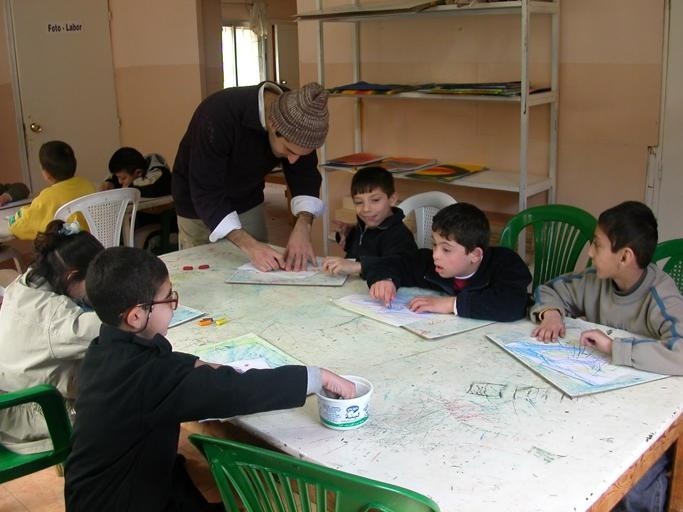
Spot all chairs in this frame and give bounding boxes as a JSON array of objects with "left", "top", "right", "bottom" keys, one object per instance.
[
  {"left": 53, "top": 187, "right": 141, "bottom": 250},
  {"left": 395, "top": 190, "right": 459, "bottom": 250},
  {"left": 0, "top": 383, "right": 73, "bottom": 486},
  {"left": 500, "top": 204, "right": 599, "bottom": 299},
  {"left": 187, "top": 434, "right": 440, "bottom": 512},
  {"left": 649, "top": 236, "right": 683, "bottom": 294}
]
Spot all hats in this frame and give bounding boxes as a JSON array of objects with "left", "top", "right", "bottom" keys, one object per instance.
[{"left": 268, "top": 82, "right": 329, "bottom": 149}]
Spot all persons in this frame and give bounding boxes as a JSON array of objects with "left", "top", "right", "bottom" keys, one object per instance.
[
  {"left": 172, "top": 79, "right": 330, "bottom": 272},
  {"left": 321, "top": 166, "right": 421, "bottom": 277},
  {"left": 365, "top": 202, "right": 533, "bottom": 323},
  {"left": 0, "top": 182, "right": 29, "bottom": 207},
  {"left": 64, "top": 246, "right": 357, "bottom": 512},
  {"left": 8, "top": 140, "right": 97, "bottom": 241},
  {"left": 0, "top": 219, "right": 106, "bottom": 455},
  {"left": 529, "top": 200, "right": 683, "bottom": 375},
  {"left": 100, "top": 146, "right": 178, "bottom": 250}
]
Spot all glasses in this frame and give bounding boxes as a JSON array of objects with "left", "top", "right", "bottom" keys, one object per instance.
[{"left": 121, "top": 292, "right": 179, "bottom": 311}]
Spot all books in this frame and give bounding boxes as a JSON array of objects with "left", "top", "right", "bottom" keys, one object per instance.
[
  {"left": 406, "top": 160, "right": 488, "bottom": 181},
  {"left": 354, "top": 157, "right": 437, "bottom": 174},
  {"left": 325, "top": 79, "right": 418, "bottom": 96},
  {"left": 431, "top": 81, "right": 530, "bottom": 97},
  {"left": 326, "top": 152, "right": 388, "bottom": 167}
]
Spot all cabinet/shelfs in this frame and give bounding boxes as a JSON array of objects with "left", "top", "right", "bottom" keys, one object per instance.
[{"left": 288, "top": 0, "right": 561, "bottom": 266}]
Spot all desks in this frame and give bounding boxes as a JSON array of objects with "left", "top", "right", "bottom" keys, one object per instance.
[
  {"left": 0, "top": 194, "right": 178, "bottom": 274},
  {"left": 153, "top": 239, "right": 683, "bottom": 512}
]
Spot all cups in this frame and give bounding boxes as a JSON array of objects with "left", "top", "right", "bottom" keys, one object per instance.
[{"left": 315, "top": 375, "right": 374, "bottom": 432}]
